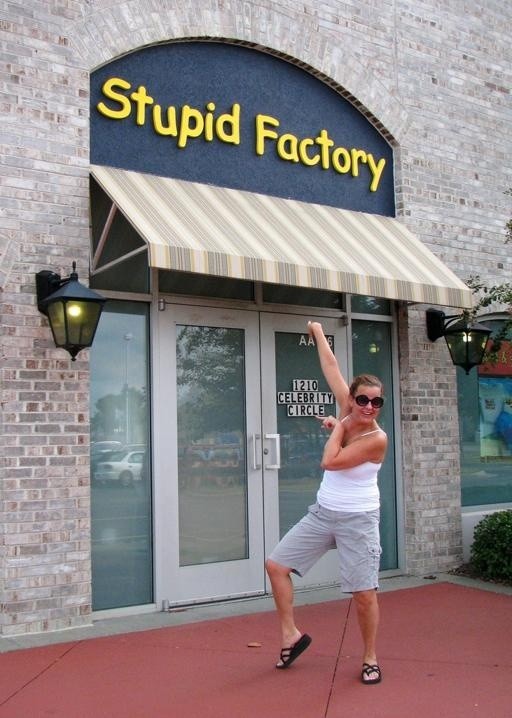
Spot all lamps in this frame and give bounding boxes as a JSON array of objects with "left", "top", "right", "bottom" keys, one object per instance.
[
  {"left": 426, "top": 303, "right": 493, "bottom": 376},
  {"left": 34, "top": 259, "right": 108, "bottom": 361}
]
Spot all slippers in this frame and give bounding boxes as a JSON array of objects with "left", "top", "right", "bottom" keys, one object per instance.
[
  {"left": 361, "top": 660, "right": 382, "bottom": 685},
  {"left": 274, "top": 632, "right": 312, "bottom": 669}
]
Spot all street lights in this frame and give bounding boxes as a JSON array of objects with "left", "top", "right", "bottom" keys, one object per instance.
[{"left": 124, "top": 332, "right": 133, "bottom": 441}]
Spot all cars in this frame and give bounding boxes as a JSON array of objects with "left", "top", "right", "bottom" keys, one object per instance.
[{"left": 91, "top": 441, "right": 143, "bottom": 487}]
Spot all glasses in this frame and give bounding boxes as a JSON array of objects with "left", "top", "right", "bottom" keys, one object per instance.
[{"left": 350, "top": 394, "right": 386, "bottom": 410}]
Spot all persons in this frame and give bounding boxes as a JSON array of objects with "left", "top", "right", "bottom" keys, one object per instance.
[{"left": 264, "top": 317, "right": 388, "bottom": 686}]
